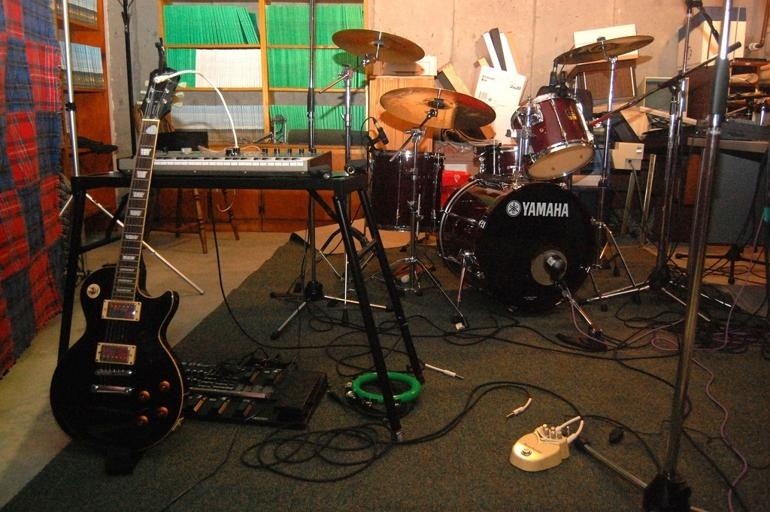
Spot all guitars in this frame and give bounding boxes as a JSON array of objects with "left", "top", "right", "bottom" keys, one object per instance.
[{"left": 50, "top": 66, "right": 185, "bottom": 455}]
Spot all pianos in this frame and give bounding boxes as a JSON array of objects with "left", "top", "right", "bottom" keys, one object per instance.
[{"left": 116, "top": 146, "right": 333, "bottom": 176}]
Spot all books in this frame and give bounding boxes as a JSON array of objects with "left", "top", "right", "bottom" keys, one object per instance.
[
  {"left": 163, "top": 2, "right": 367, "bottom": 144},
  {"left": 56, "top": 0, "right": 105, "bottom": 89}
]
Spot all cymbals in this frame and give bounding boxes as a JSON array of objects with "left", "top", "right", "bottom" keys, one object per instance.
[
  {"left": 332, "top": 29, "right": 424, "bottom": 64},
  {"left": 554, "top": 35, "right": 654, "bottom": 64},
  {"left": 380, "top": 87, "right": 496, "bottom": 129}
]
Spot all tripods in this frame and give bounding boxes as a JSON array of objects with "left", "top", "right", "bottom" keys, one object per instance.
[
  {"left": 580, "top": 1, "right": 747, "bottom": 318},
  {"left": 675, "top": 145, "right": 770, "bottom": 286},
  {"left": 363, "top": 127, "right": 469, "bottom": 331},
  {"left": 581, "top": 74, "right": 714, "bottom": 326},
  {"left": 571, "top": 1, "right": 770, "bottom": 512},
  {"left": 269, "top": 1, "right": 393, "bottom": 339},
  {"left": 327, "top": 63, "right": 359, "bottom": 326},
  {"left": 591, "top": 55, "right": 642, "bottom": 305}
]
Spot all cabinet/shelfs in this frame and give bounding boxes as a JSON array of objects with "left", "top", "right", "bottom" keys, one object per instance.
[{"left": 50, "top": 0, "right": 119, "bottom": 247}]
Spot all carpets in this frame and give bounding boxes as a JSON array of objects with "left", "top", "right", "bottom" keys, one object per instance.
[{"left": 0, "top": 211, "right": 769, "bottom": 512}]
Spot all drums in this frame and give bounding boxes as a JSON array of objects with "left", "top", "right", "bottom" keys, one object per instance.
[
  {"left": 367, "top": 149, "right": 444, "bottom": 233},
  {"left": 506, "top": 93, "right": 596, "bottom": 181},
  {"left": 476, "top": 145, "right": 520, "bottom": 182},
  {"left": 436, "top": 172, "right": 599, "bottom": 312}
]
